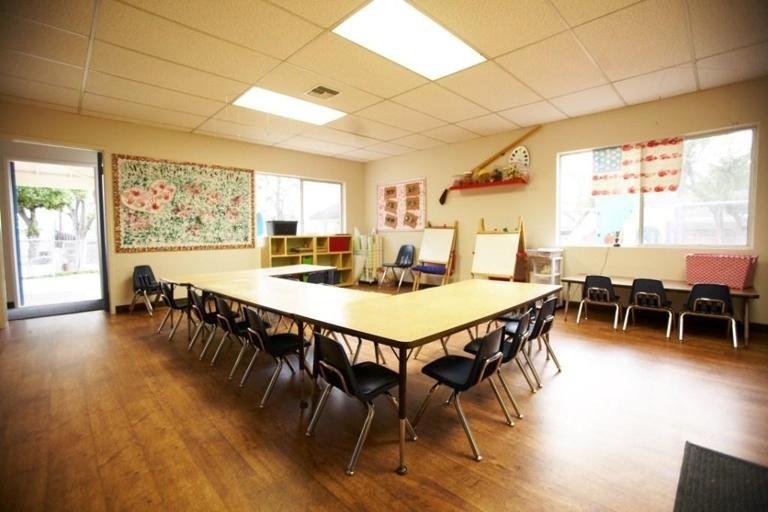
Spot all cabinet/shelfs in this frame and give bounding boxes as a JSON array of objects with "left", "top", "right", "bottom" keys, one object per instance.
[
  {"left": 529, "top": 255, "right": 563, "bottom": 309},
  {"left": 260, "top": 234, "right": 354, "bottom": 287}
]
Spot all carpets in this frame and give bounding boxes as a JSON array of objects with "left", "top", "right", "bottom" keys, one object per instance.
[{"left": 673, "top": 440, "right": 768, "bottom": 512}]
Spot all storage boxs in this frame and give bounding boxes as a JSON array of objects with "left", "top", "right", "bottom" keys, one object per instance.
[
  {"left": 266, "top": 221, "right": 298, "bottom": 235},
  {"left": 686, "top": 253, "right": 759, "bottom": 290}
]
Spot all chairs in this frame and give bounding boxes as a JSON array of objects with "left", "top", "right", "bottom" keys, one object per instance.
[
  {"left": 576, "top": 276, "right": 623, "bottom": 329},
  {"left": 129, "top": 265, "right": 162, "bottom": 317},
  {"left": 378, "top": 245, "right": 415, "bottom": 291},
  {"left": 623, "top": 278, "right": 676, "bottom": 338},
  {"left": 679, "top": 283, "right": 738, "bottom": 348}
]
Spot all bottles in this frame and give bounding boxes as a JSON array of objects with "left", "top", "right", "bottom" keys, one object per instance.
[{"left": 464, "top": 172, "right": 472, "bottom": 183}]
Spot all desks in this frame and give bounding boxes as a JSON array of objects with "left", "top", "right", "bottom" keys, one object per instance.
[
  {"left": 159, "top": 264, "right": 337, "bottom": 329},
  {"left": 562, "top": 274, "right": 760, "bottom": 347}
]
